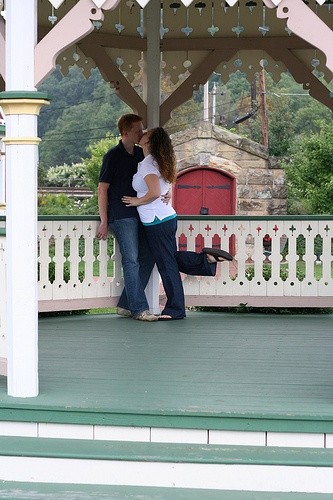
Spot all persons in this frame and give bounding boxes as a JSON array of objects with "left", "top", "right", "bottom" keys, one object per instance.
[
  {"left": 96, "top": 114, "right": 171, "bottom": 325},
  {"left": 121, "top": 127, "right": 232, "bottom": 322}
]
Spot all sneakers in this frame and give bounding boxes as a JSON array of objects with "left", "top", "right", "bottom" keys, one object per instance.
[
  {"left": 133, "top": 310, "right": 158, "bottom": 321},
  {"left": 116, "top": 306, "right": 132, "bottom": 316}
]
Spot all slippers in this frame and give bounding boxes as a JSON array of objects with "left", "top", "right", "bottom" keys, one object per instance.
[
  {"left": 156, "top": 314, "right": 184, "bottom": 320},
  {"left": 202, "top": 248, "right": 233, "bottom": 262}
]
